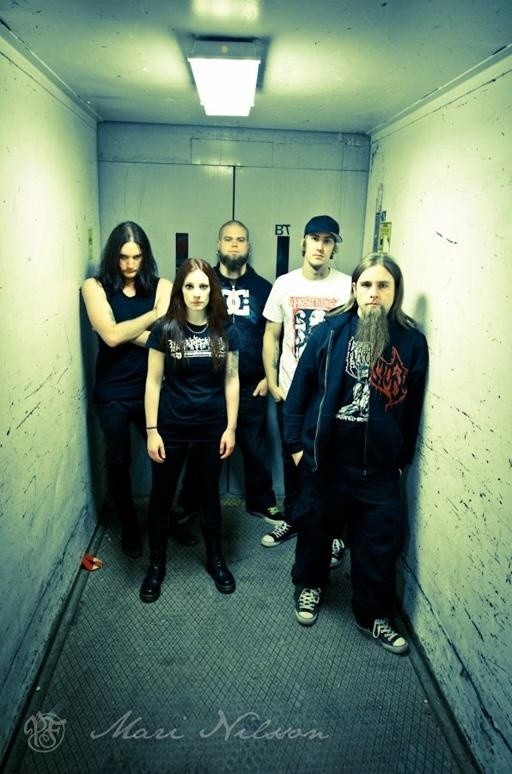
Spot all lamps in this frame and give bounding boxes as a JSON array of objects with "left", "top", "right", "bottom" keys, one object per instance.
[{"left": 186, "top": 42, "right": 260, "bottom": 120}]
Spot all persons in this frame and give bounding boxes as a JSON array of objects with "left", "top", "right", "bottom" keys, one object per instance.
[
  {"left": 78, "top": 219, "right": 201, "bottom": 558},
  {"left": 175, "top": 218, "right": 283, "bottom": 526},
  {"left": 138, "top": 257, "right": 243, "bottom": 603},
  {"left": 257, "top": 215, "right": 353, "bottom": 567},
  {"left": 280, "top": 251, "right": 430, "bottom": 655}
]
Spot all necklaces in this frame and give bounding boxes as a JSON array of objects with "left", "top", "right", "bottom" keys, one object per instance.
[{"left": 184, "top": 320, "right": 209, "bottom": 334}]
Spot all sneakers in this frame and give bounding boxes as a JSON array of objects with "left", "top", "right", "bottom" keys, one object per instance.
[
  {"left": 248, "top": 505, "right": 284, "bottom": 525},
  {"left": 294, "top": 585, "right": 322, "bottom": 626},
  {"left": 330, "top": 539, "right": 344, "bottom": 567},
  {"left": 355, "top": 617, "right": 408, "bottom": 654},
  {"left": 261, "top": 522, "right": 297, "bottom": 548}
]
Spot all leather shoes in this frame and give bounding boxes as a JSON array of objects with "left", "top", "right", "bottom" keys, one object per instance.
[
  {"left": 123, "top": 524, "right": 143, "bottom": 557},
  {"left": 141, "top": 564, "right": 166, "bottom": 602},
  {"left": 168, "top": 524, "right": 199, "bottom": 546},
  {"left": 204, "top": 554, "right": 235, "bottom": 594}
]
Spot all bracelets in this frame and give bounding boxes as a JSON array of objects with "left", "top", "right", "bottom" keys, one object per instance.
[{"left": 146, "top": 427, "right": 158, "bottom": 430}]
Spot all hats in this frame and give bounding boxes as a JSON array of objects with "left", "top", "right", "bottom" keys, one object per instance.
[{"left": 304, "top": 216, "right": 343, "bottom": 243}]
